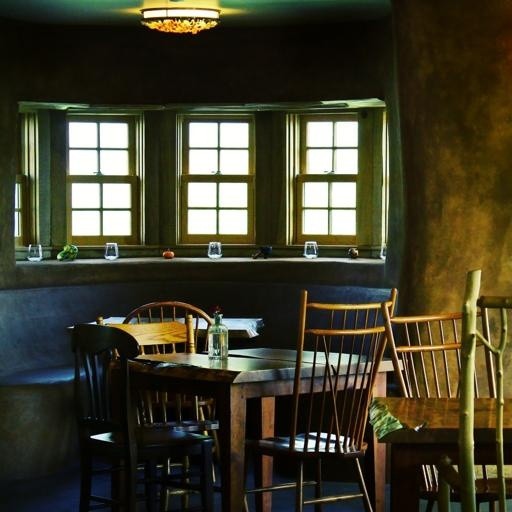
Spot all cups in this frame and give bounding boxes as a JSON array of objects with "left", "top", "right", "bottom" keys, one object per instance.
[
  {"left": 207, "top": 242, "right": 223, "bottom": 259},
  {"left": 302, "top": 240, "right": 321, "bottom": 259},
  {"left": 103, "top": 242, "right": 121, "bottom": 260},
  {"left": 27, "top": 244, "right": 44, "bottom": 262}
]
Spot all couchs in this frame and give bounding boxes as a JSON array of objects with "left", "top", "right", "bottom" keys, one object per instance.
[{"left": 4, "top": 272, "right": 398, "bottom": 489}]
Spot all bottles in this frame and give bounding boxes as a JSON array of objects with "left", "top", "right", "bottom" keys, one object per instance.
[{"left": 208, "top": 314, "right": 228, "bottom": 360}]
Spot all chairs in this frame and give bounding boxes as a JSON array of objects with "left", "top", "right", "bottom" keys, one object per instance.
[
  {"left": 370, "top": 285, "right": 512, "bottom": 511},
  {"left": 63, "top": 319, "right": 216, "bottom": 509},
  {"left": 240, "top": 287, "right": 397, "bottom": 512},
  {"left": 121, "top": 295, "right": 228, "bottom": 512},
  {"left": 91, "top": 312, "right": 222, "bottom": 512}
]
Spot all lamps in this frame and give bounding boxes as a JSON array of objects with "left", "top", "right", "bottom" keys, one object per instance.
[{"left": 132, "top": 2, "right": 225, "bottom": 40}]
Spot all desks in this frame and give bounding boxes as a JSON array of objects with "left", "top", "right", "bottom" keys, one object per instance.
[
  {"left": 367, "top": 393, "right": 512, "bottom": 510},
  {"left": 122, "top": 344, "right": 402, "bottom": 512},
  {"left": 80, "top": 310, "right": 267, "bottom": 348}
]
